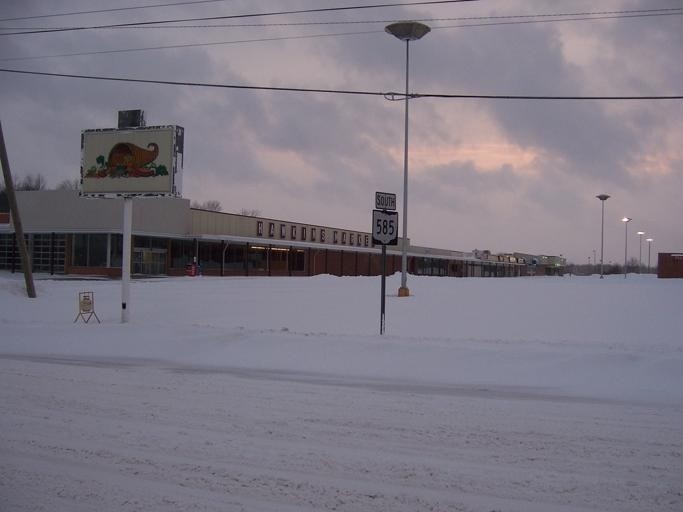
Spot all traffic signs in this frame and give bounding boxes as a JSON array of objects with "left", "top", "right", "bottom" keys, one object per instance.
[{"left": 374, "top": 192, "right": 397, "bottom": 211}]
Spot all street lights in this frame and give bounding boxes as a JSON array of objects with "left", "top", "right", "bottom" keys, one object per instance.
[
  {"left": 384, "top": 20, "right": 431, "bottom": 298},
  {"left": 594, "top": 193, "right": 653, "bottom": 279}
]
